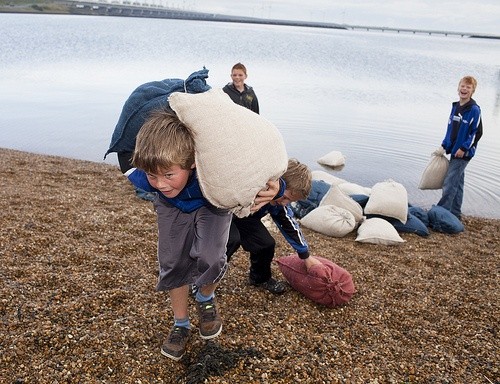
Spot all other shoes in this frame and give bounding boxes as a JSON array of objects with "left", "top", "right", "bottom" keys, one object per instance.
[{"left": 248, "top": 275, "right": 286, "bottom": 295}]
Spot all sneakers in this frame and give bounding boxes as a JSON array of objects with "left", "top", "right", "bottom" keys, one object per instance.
[
  {"left": 161, "top": 325, "right": 193, "bottom": 361},
  {"left": 193, "top": 295, "right": 223, "bottom": 339}
]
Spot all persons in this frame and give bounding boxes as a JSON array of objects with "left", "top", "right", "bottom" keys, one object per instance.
[
  {"left": 223, "top": 63, "right": 260, "bottom": 114},
  {"left": 119, "top": 113, "right": 286, "bottom": 361},
  {"left": 191, "top": 158, "right": 324, "bottom": 301},
  {"left": 436, "top": 75, "right": 484, "bottom": 220}
]
